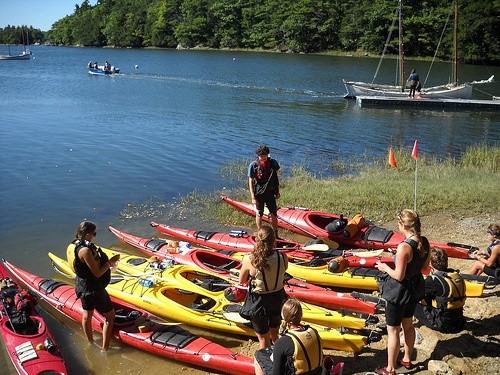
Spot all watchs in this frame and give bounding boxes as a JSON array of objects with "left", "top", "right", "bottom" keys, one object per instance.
[{"left": 108, "top": 261, "right": 112, "bottom": 265}]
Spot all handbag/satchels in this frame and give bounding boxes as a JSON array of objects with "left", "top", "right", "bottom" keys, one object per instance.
[
  {"left": 377, "top": 273, "right": 417, "bottom": 307},
  {"left": 253, "top": 181, "right": 272, "bottom": 199}
]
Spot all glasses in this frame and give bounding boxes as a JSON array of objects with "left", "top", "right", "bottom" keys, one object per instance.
[{"left": 92, "top": 232, "right": 97, "bottom": 237}]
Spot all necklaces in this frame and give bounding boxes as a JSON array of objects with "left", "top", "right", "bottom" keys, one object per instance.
[{"left": 408, "top": 234, "right": 414, "bottom": 238}]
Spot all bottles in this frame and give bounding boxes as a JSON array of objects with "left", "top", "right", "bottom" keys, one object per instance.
[{"left": 139, "top": 278, "right": 154, "bottom": 287}]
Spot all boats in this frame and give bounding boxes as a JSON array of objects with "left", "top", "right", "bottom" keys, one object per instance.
[
  {"left": 2, "top": 258, "right": 257, "bottom": 375},
  {"left": 220, "top": 196, "right": 473, "bottom": 260},
  {"left": 0, "top": 262, "right": 68, "bottom": 375},
  {"left": 0, "top": 52, "right": 31, "bottom": 59},
  {"left": 221, "top": 250, "right": 485, "bottom": 297},
  {"left": 96, "top": 244, "right": 371, "bottom": 330},
  {"left": 88, "top": 65, "right": 121, "bottom": 75},
  {"left": 151, "top": 221, "right": 396, "bottom": 269},
  {"left": 107, "top": 225, "right": 378, "bottom": 315},
  {"left": 48, "top": 252, "right": 371, "bottom": 352}
]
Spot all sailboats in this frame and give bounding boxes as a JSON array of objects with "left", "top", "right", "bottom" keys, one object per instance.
[{"left": 342, "top": 1, "right": 473, "bottom": 99}]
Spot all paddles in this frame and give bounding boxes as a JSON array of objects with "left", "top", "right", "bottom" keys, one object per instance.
[
  {"left": 0, "top": 295, "right": 16, "bottom": 333},
  {"left": 114, "top": 314, "right": 183, "bottom": 325},
  {"left": 277, "top": 216, "right": 339, "bottom": 249},
  {"left": 294, "top": 249, "right": 385, "bottom": 265},
  {"left": 203, "top": 263, "right": 239, "bottom": 276},
  {"left": 273, "top": 244, "right": 330, "bottom": 251},
  {"left": 192, "top": 308, "right": 251, "bottom": 323},
  {"left": 213, "top": 283, "right": 249, "bottom": 290}
]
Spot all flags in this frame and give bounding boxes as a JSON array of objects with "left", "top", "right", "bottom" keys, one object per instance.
[
  {"left": 410, "top": 140, "right": 418, "bottom": 159},
  {"left": 389, "top": 147, "right": 397, "bottom": 168}
]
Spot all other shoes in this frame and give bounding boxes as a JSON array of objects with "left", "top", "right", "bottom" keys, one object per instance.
[
  {"left": 374, "top": 367, "right": 395, "bottom": 375},
  {"left": 398, "top": 357, "right": 411, "bottom": 369}
]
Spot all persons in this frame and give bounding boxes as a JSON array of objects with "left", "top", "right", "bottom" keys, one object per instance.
[
  {"left": 238, "top": 225, "right": 288, "bottom": 349},
  {"left": 375, "top": 209, "right": 431, "bottom": 375},
  {"left": 406, "top": 68, "right": 419, "bottom": 99},
  {"left": 254, "top": 299, "right": 324, "bottom": 375},
  {"left": 66, "top": 221, "right": 120, "bottom": 351},
  {"left": 93, "top": 61, "right": 98, "bottom": 69},
  {"left": 413, "top": 246, "right": 466, "bottom": 332},
  {"left": 104, "top": 61, "right": 112, "bottom": 74},
  {"left": 463, "top": 222, "right": 500, "bottom": 279},
  {"left": 88, "top": 61, "right": 94, "bottom": 68},
  {"left": 247, "top": 146, "right": 284, "bottom": 240}
]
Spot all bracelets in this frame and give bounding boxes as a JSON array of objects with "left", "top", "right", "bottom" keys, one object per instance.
[{"left": 477, "top": 256, "right": 481, "bottom": 260}]
